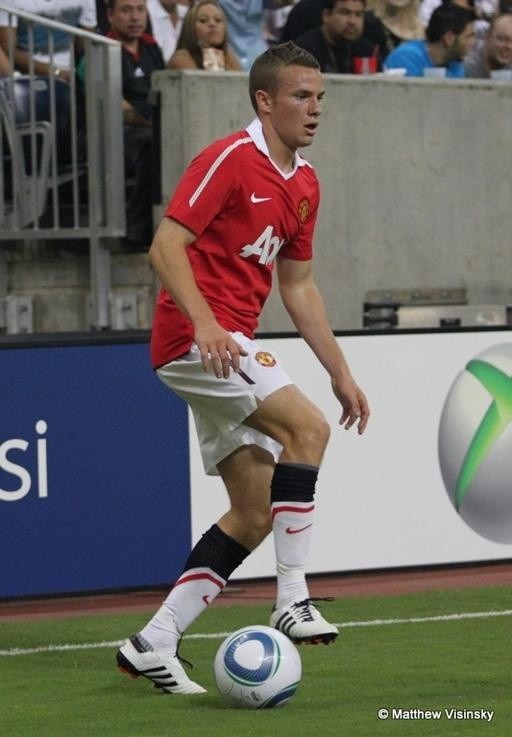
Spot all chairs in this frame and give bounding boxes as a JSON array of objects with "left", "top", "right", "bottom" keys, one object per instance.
[{"left": 0, "top": 76, "right": 140, "bottom": 230}]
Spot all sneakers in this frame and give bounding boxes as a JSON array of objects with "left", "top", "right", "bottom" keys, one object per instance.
[
  {"left": 266, "top": 601, "right": 339, "bottom": 645},
  {"left": 114, "top": 629, "right": 208, "bottom": 695}
]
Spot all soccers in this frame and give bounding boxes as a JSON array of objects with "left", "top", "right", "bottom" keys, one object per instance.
[
  {"left": 212, "top": 624, "right": 303, "bottom": 708},
  {"left": 438, "top": 343, "right": 511, "bottom": 545}
]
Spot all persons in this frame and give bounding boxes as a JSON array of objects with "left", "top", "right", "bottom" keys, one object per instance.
[
  {"left": 0, "top": 0, "right": 510, "bottom": 256},
  {"left": 110, "top": 39, "right": 370, "bottom": 695}
]
